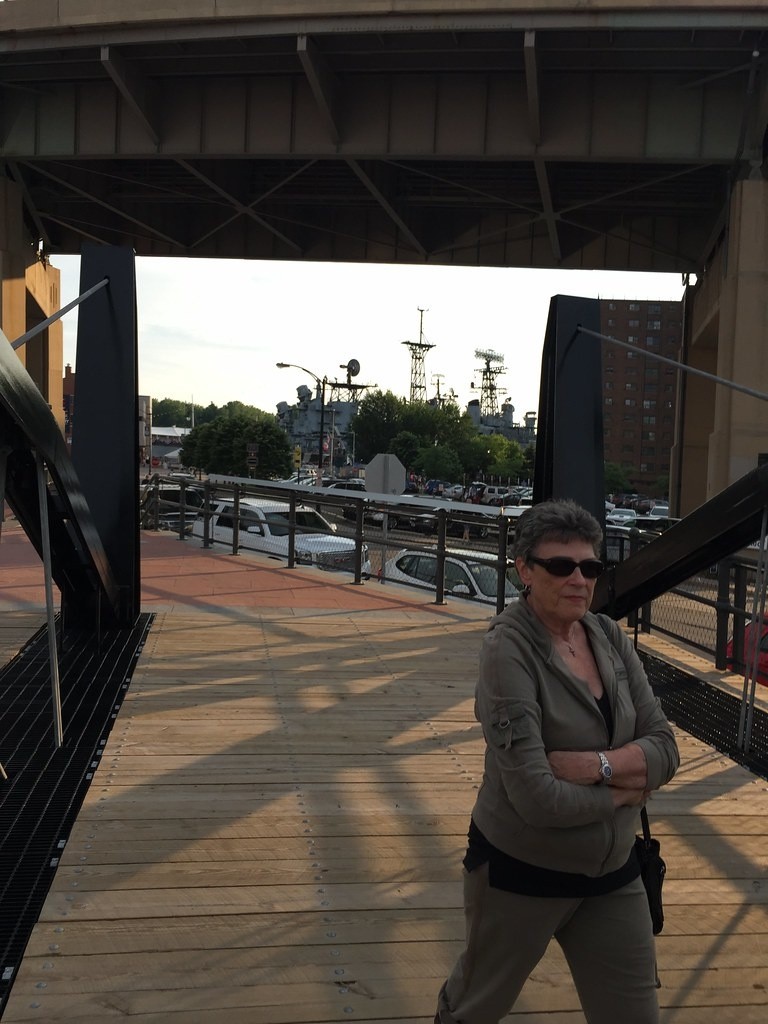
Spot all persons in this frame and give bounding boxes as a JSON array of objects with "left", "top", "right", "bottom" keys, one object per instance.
[{"left": 434, "top": 498, "right": 680, "bottom": 1024}]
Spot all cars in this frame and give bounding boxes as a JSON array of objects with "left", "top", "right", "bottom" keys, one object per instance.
[
  {"left": 726, "top": 610, "right": 768, "bottom": 688},
  {"left": 140, "top": 482, "right": 208, "bottom": 536},
  {"left": 277, "top": 465, "right": 670, "bottom": 546}
]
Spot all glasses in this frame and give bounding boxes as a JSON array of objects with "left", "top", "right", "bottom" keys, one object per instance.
[{"left": 527, "top": 556, "right": 605, "bottom": 579}]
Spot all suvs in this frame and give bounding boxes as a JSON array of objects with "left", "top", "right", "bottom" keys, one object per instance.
[
  {"left": 378, "top": 543, "right": 526, "bottom": 614},
  {"left": 190, "top": 496, "right": 373, "bottom": 581}
]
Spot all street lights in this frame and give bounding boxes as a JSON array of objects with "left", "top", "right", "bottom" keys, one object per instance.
[{"left": 277, "top": 362, "right": 326, "bottom": 515}]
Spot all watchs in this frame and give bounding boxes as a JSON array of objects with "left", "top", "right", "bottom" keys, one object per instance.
[{"left": 596, "top": 751, "right": 613, "bottom": 787}]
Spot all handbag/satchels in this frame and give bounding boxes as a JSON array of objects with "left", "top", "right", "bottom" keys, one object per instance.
[{"left": 641, "top": 839, "right": 666, "bottom": 935}]
